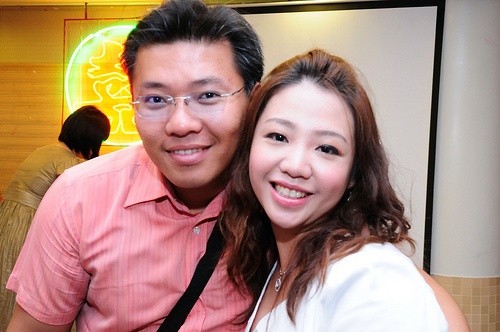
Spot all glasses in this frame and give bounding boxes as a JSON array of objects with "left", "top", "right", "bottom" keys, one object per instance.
[{"left": 130, "top": 85, "right": 251, "bottom": 119}]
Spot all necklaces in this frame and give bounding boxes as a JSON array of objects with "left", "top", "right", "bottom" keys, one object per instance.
[{"left": 272, "top": 254, "right": 306, "bottom": 292}]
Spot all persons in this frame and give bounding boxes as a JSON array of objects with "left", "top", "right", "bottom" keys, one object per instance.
[
  {"left": 218, "top": 49, "right": 450, "bottom": 331},
  {"left": 3, "top": 0, "right": 265, "bottom": 332},
  {"left": 0, "top": 103, "right": 110, "bottom": 332}
]
